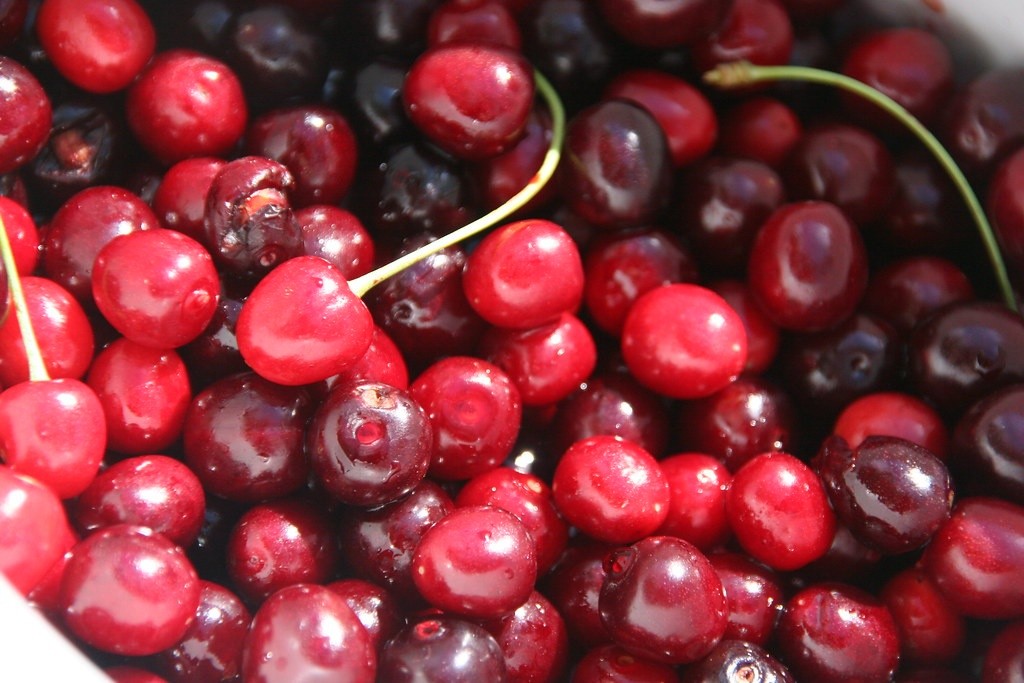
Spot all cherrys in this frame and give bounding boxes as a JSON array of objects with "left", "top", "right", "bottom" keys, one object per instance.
[{"left": 1, "top": 1, "right": 1024, "bottom": 683}]
